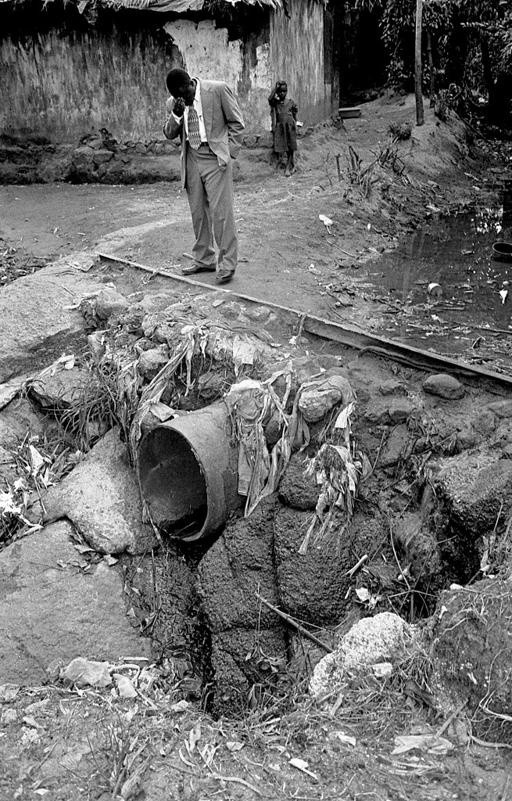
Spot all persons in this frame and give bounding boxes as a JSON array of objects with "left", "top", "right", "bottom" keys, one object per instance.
[
  {"left": 267, "top": 80, "right": 303, "bottom": 178},
  {"left": 162, "top": 67, "right": 246, "bottom": 285}
]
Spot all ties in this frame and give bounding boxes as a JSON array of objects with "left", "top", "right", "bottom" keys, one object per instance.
[{"left": 188, "top": 104, "right": 202, "bottom": 150}]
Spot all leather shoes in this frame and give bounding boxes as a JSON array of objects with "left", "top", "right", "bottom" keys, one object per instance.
[
  {"left": 181, "top": 264, "right": 216, "bottom": 274},
  {"left": 215, "top": 269, "right": 235, "bottom": 279}
]
